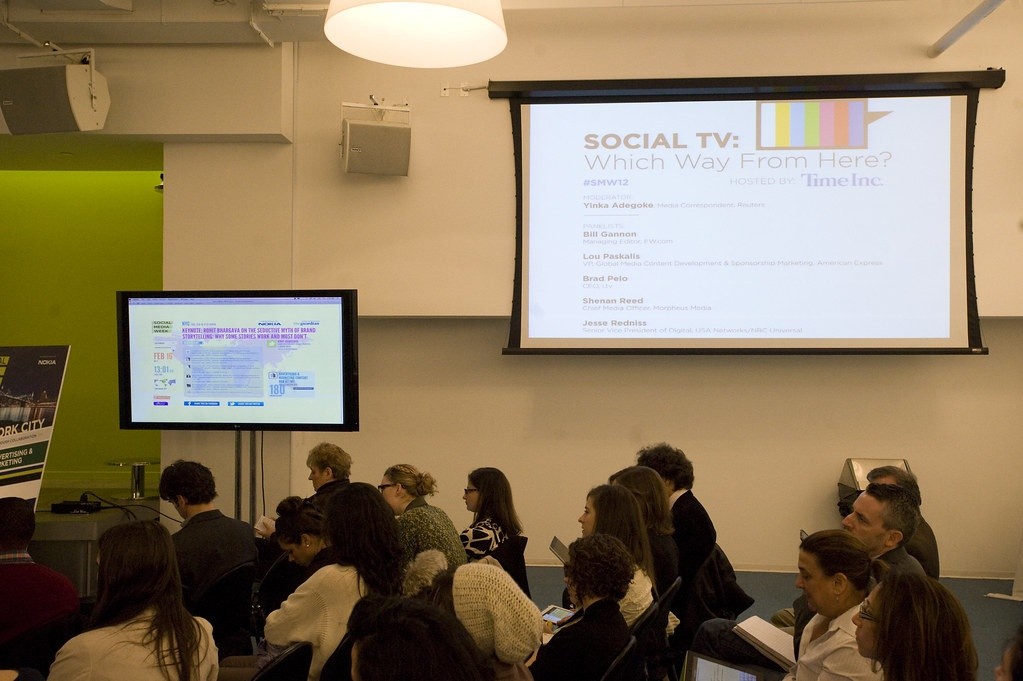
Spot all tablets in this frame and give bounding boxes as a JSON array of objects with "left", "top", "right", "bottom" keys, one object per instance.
[
  {"left": 549, "top": 536, "right": 571, "bottom": 564},
  {"left": 684, "top": 650, "right": 764, "bottom": 681},
  {"left": 541, "top": 605, "right": 573, "bottom": 623}
]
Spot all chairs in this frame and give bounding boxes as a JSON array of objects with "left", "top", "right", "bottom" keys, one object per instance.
[
  {"left": 492, "top": 536, "right": 529, "bottom": 574},
  {"left": 251, "top": 641, "right": 314, "bottom": 681},
  {"left": 601, "top": 576, "right": 681, "bottom": 681},
  {"left": 189, "top": 562, "right": 256, "bottom": 656},
  {"left": 251, "top": 549, "right": 306, "bottom": 639},
  {"left": 0, "top": 607, "right": 82, "bottom": 681},
  {"left": 320, "top": 633, "right": 351, "bottom": 681}
]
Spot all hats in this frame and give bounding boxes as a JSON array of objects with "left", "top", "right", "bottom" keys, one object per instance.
[{"left": 452, "top": 562, "right": 545, "bottom": 666}]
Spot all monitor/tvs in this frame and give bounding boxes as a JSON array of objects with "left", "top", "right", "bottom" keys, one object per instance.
[{"left": 116, "top": 289, "right": 360, "bottom": 433}]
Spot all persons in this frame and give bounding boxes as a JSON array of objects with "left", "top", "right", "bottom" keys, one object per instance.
[{"left": 0, "top": 442, "right": 1022, "bottom": 680}]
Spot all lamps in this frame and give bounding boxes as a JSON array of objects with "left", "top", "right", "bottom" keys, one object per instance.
[{"left": 324, "top": 0, "right": 508, "bottom": 68}]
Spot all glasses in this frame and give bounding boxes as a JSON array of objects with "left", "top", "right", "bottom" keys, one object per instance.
[
  {"left": 378, "top": 483, "right": 405, "bottom": 494},
  {"left": 464, "top": 489, "right": 477, "bottom": 495},
  {"left": 858, "top": 600, "right": 878, "bottom": 623}
]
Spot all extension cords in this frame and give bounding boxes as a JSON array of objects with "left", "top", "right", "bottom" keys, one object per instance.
[{"left": 51, "top": 501, "right": 102, "bottom": 514}]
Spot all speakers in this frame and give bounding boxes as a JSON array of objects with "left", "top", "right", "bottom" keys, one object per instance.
[
  {"left": 0, "top": 64, "right": 111, "bottom": 137},
  {"left": 341, "top": 120, "right": 411, "bottom": 176}
]
